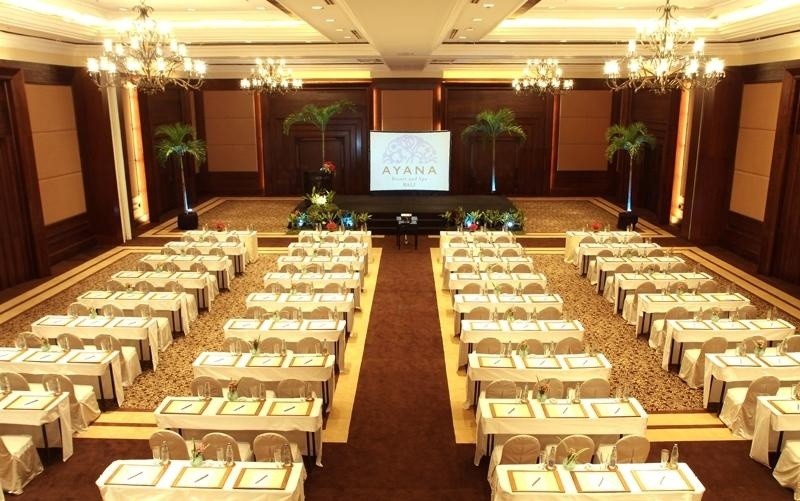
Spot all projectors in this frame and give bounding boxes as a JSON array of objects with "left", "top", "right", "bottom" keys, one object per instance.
[{"left": 400, "top": 213, "right": 412, "bottom": 224}]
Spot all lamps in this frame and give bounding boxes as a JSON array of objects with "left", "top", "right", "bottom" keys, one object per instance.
[
  {"left": 509, "top": 1, "right": 728, "bottom": 97},
  {"left": 86, "top": 1, "right": 304, "bottom": 97}
]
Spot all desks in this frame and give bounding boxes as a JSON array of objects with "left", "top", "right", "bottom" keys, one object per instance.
[{"left": 395, "top": 224, "right": 419, "bottom": 252}]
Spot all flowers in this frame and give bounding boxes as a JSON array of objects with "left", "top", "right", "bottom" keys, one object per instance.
[{"left": 318, "top": 160, "right": 337, "bottom": 177}]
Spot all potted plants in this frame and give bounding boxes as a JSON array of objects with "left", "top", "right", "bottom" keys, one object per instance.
[
  {"left": 152, "top": 123, "right": 208, "bottom": 228},
  {"left": 287, "top": 206, "right": 373, "bottom": 231},
  {"left": 440, "top": 206, "right": 526, "bottom": 231},
  {"left": 606, "top": 123, "right": 655, "bottom": 229}
]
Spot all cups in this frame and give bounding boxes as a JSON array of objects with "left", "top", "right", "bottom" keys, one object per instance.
[
  {"left": 579, "top": 221, "right": 799, "bottom": 412},
  {"left": 443, "top": 224, "right": 680, "bottom": 473},
  {"left": 149, "top": 229, "right": 370, "bottom": 468},
  {"left": 0, "top": 248, "right": 154, "bottom": 396}
]
